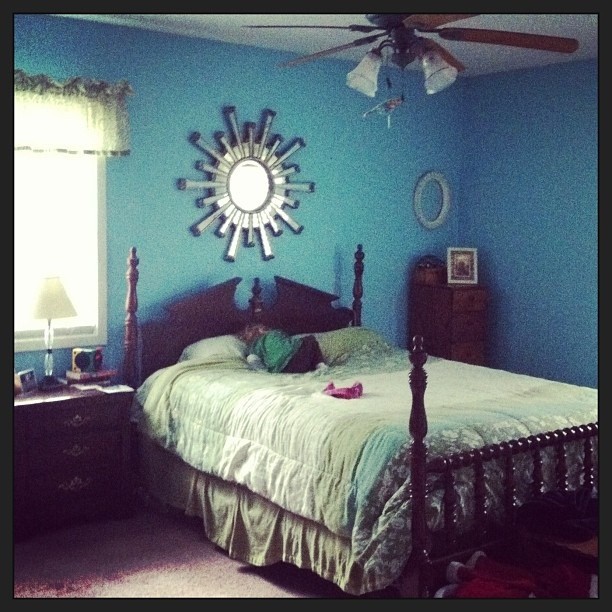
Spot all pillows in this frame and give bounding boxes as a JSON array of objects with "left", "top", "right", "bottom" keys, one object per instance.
[
  {"left": 294, "top": 327, "right": 393, "bottom": 365},
  {"left": 183, "top": 336, "right": 249, "bottom": 364}
]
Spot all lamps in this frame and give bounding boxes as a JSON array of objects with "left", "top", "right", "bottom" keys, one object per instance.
[
  {"left": 343, "top": 23, "right": 460, "bottom": 99},
  {"left": 34, "top": 275, "right": 75, "bottom": 390}
]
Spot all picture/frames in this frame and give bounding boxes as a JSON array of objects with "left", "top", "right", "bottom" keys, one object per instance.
[{"left": 444, "top": 246, "right": 480, "bottom": 287}]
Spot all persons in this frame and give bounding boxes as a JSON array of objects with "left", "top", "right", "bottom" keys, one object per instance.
[{"left": 236, "top": 320, "right": 327, "bottom": 374}]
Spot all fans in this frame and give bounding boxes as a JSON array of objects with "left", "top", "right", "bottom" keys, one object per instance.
[{"left": 239, "top": 15, "right": 580, "bottom": 72}]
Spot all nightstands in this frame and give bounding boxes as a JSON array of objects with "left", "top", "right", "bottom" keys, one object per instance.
[
  {"left": 406, "top": 276, "right": 490, "bottom": 366},
  {"left": 14, "top": 378, "right": 137, "bottom": 536}
]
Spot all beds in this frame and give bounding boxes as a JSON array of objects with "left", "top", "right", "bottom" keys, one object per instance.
[{"left": 118, "top": 242, "right": 599, "bottom": 598}]
